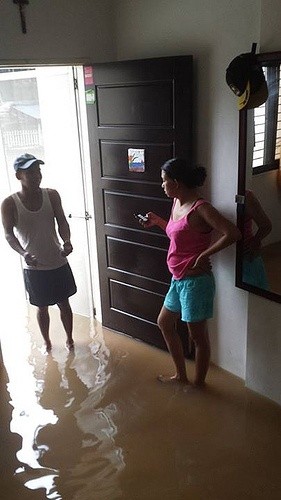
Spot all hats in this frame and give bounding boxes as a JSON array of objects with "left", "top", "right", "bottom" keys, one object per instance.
[
  {"left": 227, "top": 52, "right": 253, "bottom": 111},
  {"left": 14, "top": 153, "right": 44, "bottom": 171}
]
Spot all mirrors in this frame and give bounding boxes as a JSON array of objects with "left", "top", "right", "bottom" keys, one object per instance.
[{"left": 235, "top": 51, "right": 281, "bottom": 304}]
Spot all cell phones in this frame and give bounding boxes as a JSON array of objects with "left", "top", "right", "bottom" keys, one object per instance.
[{"left": 133, "top": 212, "right": 150, "bottom": 224}]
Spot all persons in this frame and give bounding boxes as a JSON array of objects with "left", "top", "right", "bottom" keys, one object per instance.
[
  {"left": 1, "top": 153, "right": 78, "bottom": 345},
  {"left": 241, "top": 191, "right": 273, "bottom": 291},
  {"left": 139, "top": 158, "right": 240, "bottom": 390}
]
